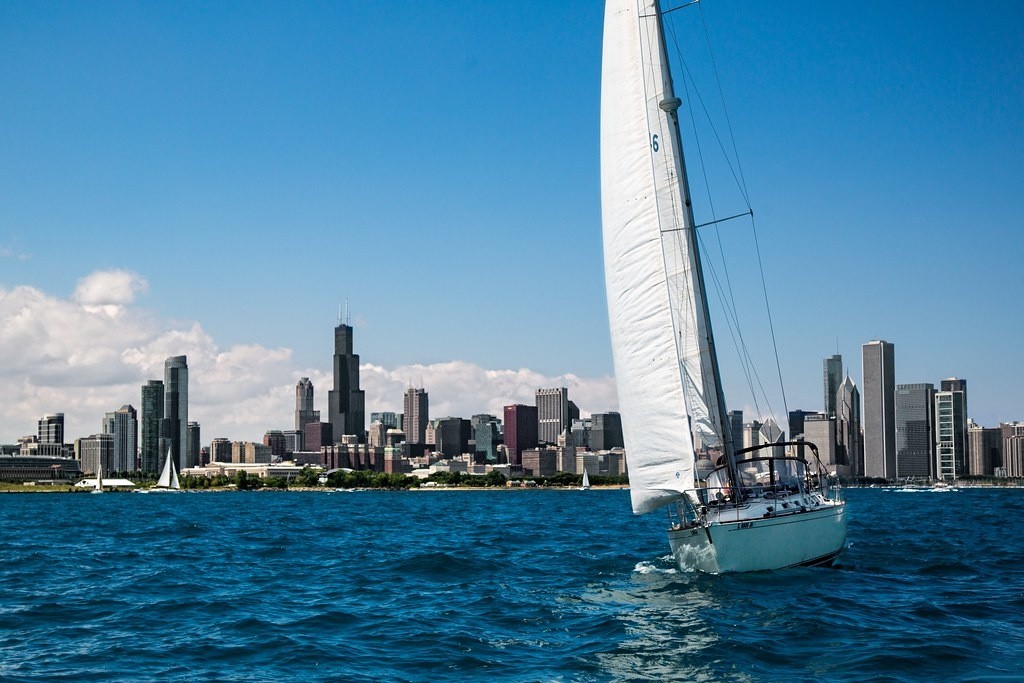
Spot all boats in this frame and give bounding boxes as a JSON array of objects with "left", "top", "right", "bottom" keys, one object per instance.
[{"left": 931, "top": 481, "right": 954, "bottom": 492}]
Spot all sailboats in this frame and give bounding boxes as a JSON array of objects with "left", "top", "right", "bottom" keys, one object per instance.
[
  {"left": 596, "top": 0, "right": 849, "bottom": 577},
  {"left": 91, "top": 463, "right": 105, "bottom": 494},
  {"left": 150, "top": 445, "right": 180, "bottom": 492},
  {"left": 580, "top": 467, "right": 591, "bottom": 491}
]
